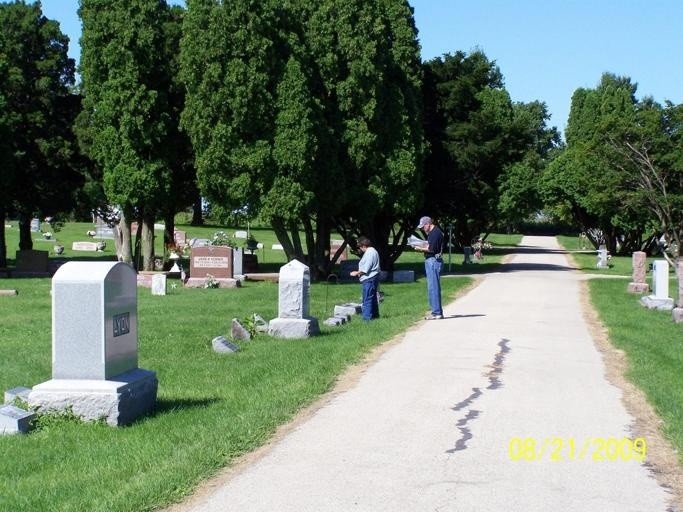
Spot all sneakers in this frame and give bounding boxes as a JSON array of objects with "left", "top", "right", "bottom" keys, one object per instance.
[{"left": 424, "top": 313, "right": 443, "bottom": 320}]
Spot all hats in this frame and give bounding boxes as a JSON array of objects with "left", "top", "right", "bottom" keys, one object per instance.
[{"left": 417, "top": 216, "right": 432, "bottom": 229}]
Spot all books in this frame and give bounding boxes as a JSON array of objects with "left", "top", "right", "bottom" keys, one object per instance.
[{"left": 405, "top": 234, "right": 427, "bottom": 249}]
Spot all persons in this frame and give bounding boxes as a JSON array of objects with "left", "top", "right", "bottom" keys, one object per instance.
[
  {"left": 412, "top": 216, "right": 446, "bottom": 321},
  {"left": 348, "top": 236, "right": 381, "bottom": 321}
]
